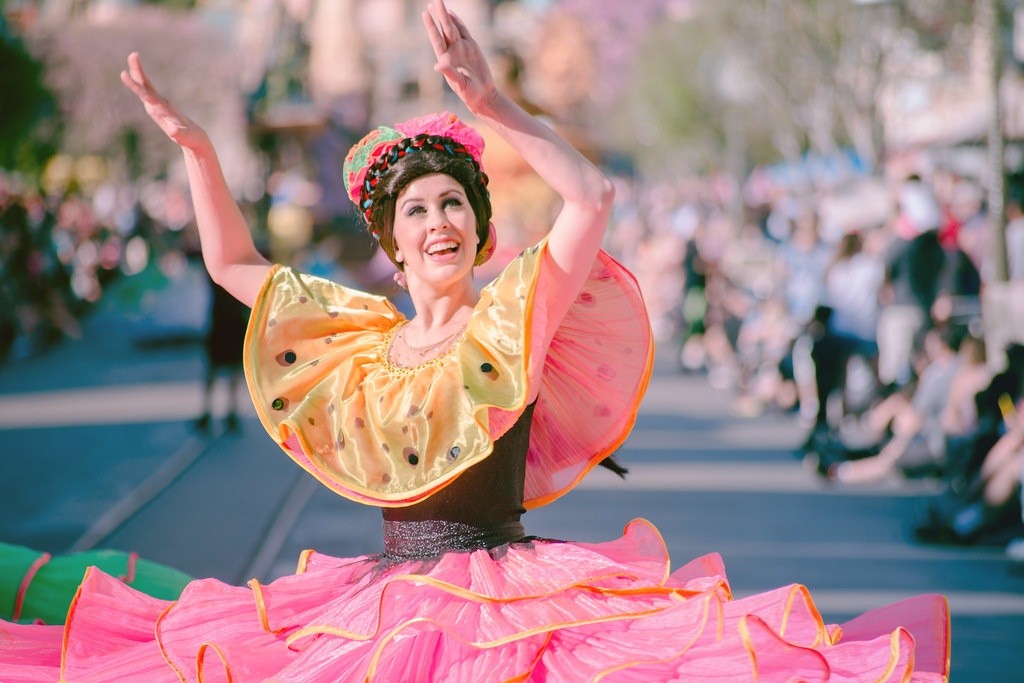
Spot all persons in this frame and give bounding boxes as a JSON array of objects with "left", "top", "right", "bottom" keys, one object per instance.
[
  {"left": 616, "top": 143, "right": 1023, "bottom": 569},
  {"left": 120, "top": 1, "right": 618, "bottom": 683},
  {"left": 2, "top": 152, "right": 275, "bottom": 437}
]
